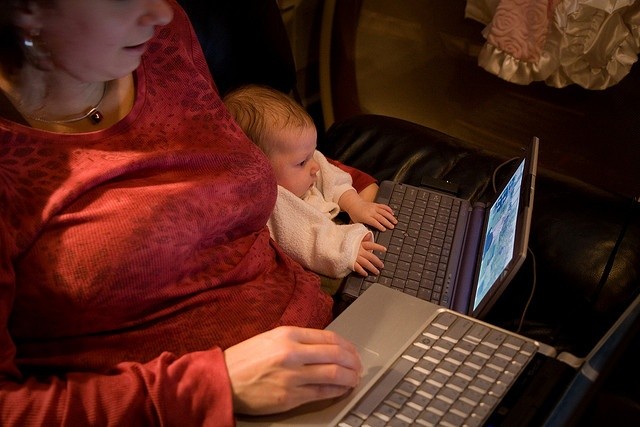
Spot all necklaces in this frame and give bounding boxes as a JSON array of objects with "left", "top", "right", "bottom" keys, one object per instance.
[{"left": 19, "top": 79, "right": 108, "bottom": 125}]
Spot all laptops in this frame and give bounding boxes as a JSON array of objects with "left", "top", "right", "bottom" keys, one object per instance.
[{"left": 340, "top": 138, "right": 539, "bottom": 319}]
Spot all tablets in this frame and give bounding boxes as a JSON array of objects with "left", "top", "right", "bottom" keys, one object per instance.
[{"left": 235, "top": 282, "right": 640, "bottom": 427}]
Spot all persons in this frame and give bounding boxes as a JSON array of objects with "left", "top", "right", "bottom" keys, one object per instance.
[
  {"left": 224, "top": 84, "right": 398, "bottom": 279},
  {"left": 0, "top": 1, "right": 379, "bottom": 426}
]
